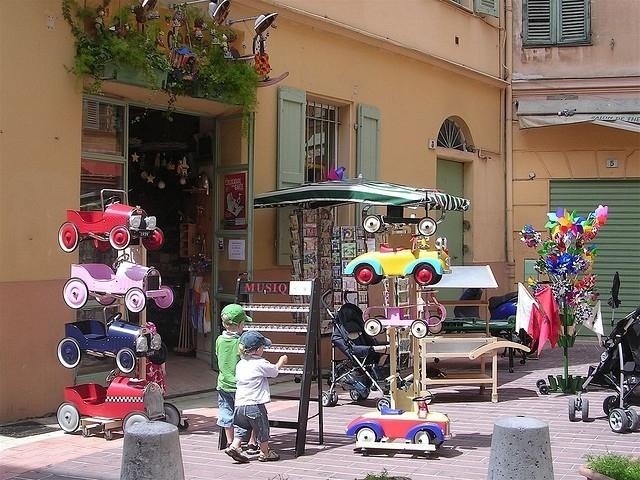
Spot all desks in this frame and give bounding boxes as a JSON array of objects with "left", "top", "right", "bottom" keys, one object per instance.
[{"left": 441, "top": 316, "right": 527, "bottom": 373}]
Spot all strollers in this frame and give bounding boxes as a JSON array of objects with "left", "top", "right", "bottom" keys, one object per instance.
[
  {"left": 561, "top": 303, "right": 640, "bottom": 436},
  {"left": 319, "top": 288, "right": 438, "bottom": 415}
]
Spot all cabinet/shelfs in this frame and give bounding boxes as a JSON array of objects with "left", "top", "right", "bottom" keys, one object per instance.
[{"left": 179, "top": 224, "right": 198, "bottom": 257}]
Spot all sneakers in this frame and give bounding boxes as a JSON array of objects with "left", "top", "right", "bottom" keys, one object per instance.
[
  {"left": 258, "top": 449, "right": 280, "bottom": 462},
  {"left": 225, "top": 441, "right": 233, "bottom": 448},
  {"left": 224, "top": 444, "right": 250, "bottom": 464},
  {"left": 246, "top": 442, "right": 261, "bottom": 455}
]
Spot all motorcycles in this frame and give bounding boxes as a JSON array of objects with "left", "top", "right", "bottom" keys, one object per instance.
[{"left": 450, "top": 277, "right": 543, "bottom": 358}]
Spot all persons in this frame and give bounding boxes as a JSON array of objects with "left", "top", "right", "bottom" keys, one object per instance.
[
  {"left": 224, "top": 330, "right": 287, "bottom": 462},
  {"left": 215, "top": 304, "right": 257, "bottom": 449}
]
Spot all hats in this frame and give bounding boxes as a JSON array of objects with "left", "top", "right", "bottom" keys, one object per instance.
[
  {"left": 221, "top": 303, "right": 253, "bottom": 325},
  {"left": 239, "top": 330, "right": 273, "bottom": 351}
]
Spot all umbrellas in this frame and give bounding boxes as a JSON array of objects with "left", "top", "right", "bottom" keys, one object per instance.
[{"left": 256, "top": 172, "right": 470, "bottom": 228}]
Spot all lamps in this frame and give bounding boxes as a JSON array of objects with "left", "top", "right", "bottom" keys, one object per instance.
[{"left": 139, "top": 0, "right": 289, "bottom": 62}]
